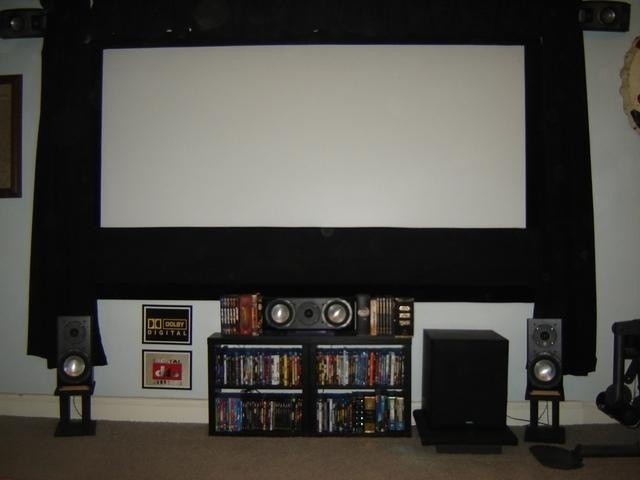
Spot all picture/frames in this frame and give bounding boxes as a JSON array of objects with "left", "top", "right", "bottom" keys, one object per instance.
[
  {"left": 0, "top": 75, "right": 22, "bottom": 198},
  {"left": 142, "top": 305, "right": 193, "bottom": 390}
]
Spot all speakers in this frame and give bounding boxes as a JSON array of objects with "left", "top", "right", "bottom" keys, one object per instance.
[
  {"left": 527, "top": 317, "right": 561, "bottom": 391},
  {"left": 423, "top": 329, "right": 509, "bottom": 433},
  {"left": 262, "top": 297, "right": 358, "bottom": 335},
  {"left": 57, "top": 315, "right": 92, "bottom": 385}
]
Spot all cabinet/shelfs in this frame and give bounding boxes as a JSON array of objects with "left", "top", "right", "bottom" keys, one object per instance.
[{"left": 208, "top": 332, "right": 412, "bottom": 437}]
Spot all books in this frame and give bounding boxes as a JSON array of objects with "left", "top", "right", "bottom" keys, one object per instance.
[
  {"left": 213, "top": 349, "right": 406, "bottom": 434},
  {"left": 218, "top": 292, "right": 262, "bottom": 336},
  {"left": 357, "top": 292, "right": 416, "bottom": 339}
]
